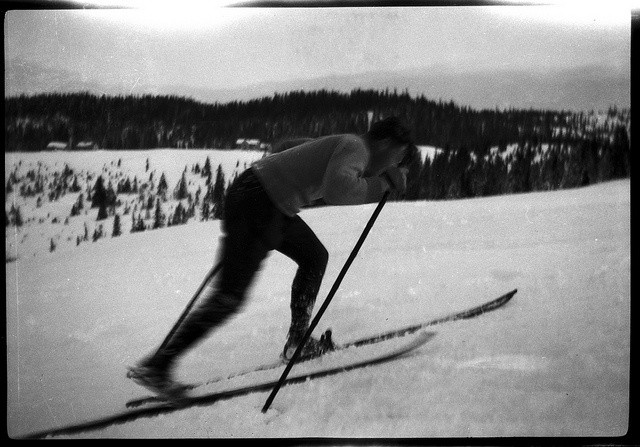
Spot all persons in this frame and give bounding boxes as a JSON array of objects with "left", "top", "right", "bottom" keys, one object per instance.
[{"left": 125, "top": 117, "right": 414, "bottom": 401}]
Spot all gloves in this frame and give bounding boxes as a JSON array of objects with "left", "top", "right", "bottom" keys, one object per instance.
[{"left": 380, "top": 165, "right": 409, "bottom": 191}]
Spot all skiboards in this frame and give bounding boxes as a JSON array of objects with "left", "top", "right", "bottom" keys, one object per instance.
[{"left": 12, "top": 289, "right": 517, "bottom": 439}]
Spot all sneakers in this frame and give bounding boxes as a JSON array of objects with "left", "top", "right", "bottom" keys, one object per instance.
[
  {"left": 280, "top": 333, "right": 321, "bottom": 356},
  {"left": 128, "top": 358, "right": 188, "bottom": 400}
]
